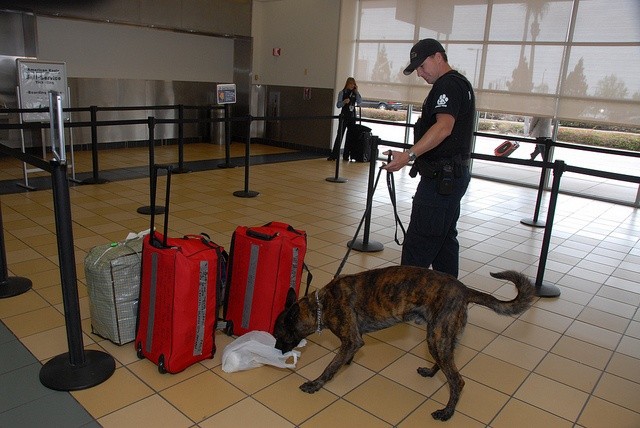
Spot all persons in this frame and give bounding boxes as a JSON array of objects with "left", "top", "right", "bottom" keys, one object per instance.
[
  {"left": 380, "top": 39, "right": 477, "bottom": 280},
  {"left": 326, "top": 76, "right": 362, "bottom": 161},
  {"left": 529, "top": 115, "right": 553, "bottom": 164}
]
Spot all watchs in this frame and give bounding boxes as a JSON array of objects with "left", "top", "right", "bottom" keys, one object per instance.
[{"left": 408, "top": 150, "right": 416, "bottom": 161}]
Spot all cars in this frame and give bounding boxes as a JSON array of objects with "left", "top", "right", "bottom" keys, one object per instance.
[{"left": 358, "top": 100, "right": 403, "bottom": 110}]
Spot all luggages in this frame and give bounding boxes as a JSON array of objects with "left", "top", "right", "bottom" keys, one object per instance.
[
  {"left": 350, "top": 106, "right": 378, "bottom": 162},
  {"left": 494, "top": 136, "right": 530, "bottom": 157}
]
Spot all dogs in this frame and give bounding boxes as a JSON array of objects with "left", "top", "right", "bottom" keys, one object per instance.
[{"left": 274, "top": 266, "right": 536, "bottom": 421}]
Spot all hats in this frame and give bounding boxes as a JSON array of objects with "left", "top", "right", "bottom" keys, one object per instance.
[{"left": 403, "top": 38, "right": 445, "bottom": 75}]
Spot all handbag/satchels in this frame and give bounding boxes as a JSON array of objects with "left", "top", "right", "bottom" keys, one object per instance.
[
  {"left": 224, "top": 221, "right": 313, "bottom": 336},
  {"left": 135, "top": 164, "right": 229, "bottom": 374},
  {"left": 84, "top": 237, "right": 157, "bottom": 346}
]
